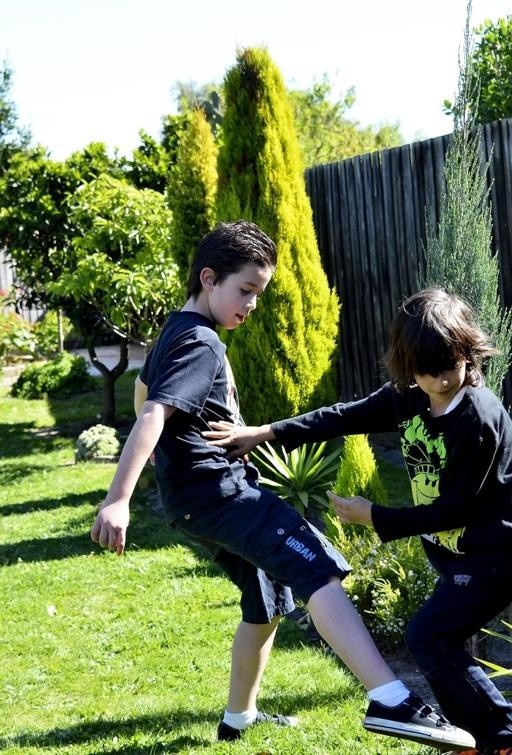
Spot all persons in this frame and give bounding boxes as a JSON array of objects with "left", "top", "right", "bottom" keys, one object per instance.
[
  {"left": 200, "top": 284, "right": 511, "bottom": 752},
  {"left": 87, "top": 215, "right": 481, "bottom": 753}
]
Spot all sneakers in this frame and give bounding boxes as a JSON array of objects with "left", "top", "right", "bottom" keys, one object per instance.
[
  {"left": 361, "top": 689, "right": 477, "bottom": 751},
  {"left": 217, "top": 709, "right": 299, "bottom": 744}
]
